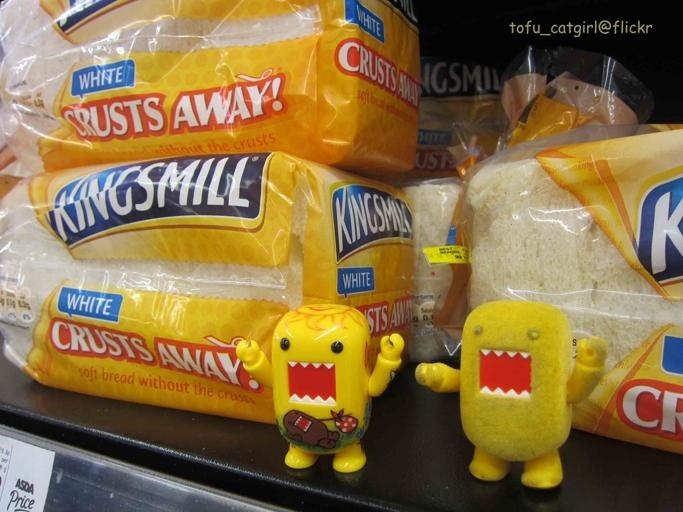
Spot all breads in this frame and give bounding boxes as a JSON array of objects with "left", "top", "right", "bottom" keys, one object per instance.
[
  {"left": 0, "top": 0, "right": 414, "bottom": 171},
  {"left": 1, "top": 152, "right": 413, "bottom": 424},
  {"left": 468, "top": 159, "right": 683, "bottom": 456},
  {"left": 401, "top": 183, "right": 469, "bottom": 361}
]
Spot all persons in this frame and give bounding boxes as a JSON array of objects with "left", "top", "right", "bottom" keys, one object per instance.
[{"left": 414, "top": 300, "right": 608, "bottom": 491}]
